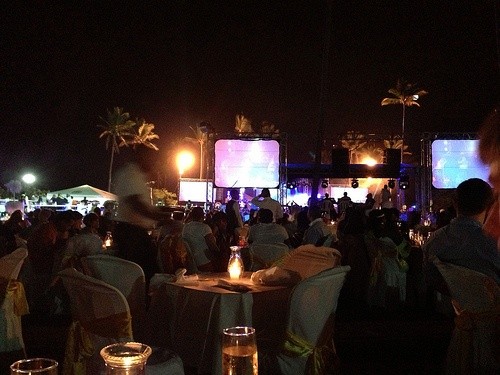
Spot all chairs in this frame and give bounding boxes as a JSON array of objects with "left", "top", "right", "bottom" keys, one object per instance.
[
  {"left": 61, "top": 267, "right": 182, "bottom": 375},
  {"left": 171, "top": 237, "right": 199, "bottom": 274},
  {"left": 0, "top": 248, "right": 27, "bottom": 360},
  {"left": 251, "top": 242, "right": 288, "bottom": 272},
  {"left": 80, "top": 254, "right": 146, "bottom": 343},
  {"left": 260, "top": 266, "right": 351, "bottom": 374}
]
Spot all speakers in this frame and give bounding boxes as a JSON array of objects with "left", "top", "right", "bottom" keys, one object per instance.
[
  {"left": 332, "top": 148, "right": 349, "bottom": 173},
  {"left": 383, "top": 148, "right": 401, "bottom": 177}
]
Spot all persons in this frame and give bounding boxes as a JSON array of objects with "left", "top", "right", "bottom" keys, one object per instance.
[
  {"left": 423, "top": 179, "right": 500, "bottom": 289},
  {"left": 445, "top": 109, "right": 500, "bottom": 374},
  {"left": 0, "top": 178, "right": 456, "bottom": 375}
]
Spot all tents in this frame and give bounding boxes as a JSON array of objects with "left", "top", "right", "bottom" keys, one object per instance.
[{"left": 46, "top": 184, "right": 118, "bottom": 207}]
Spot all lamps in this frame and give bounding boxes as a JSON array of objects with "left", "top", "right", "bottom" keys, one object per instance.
[
  {"left": 322, "top": 179, "right": 328, "bottom": 188},
  {"left": 398, "top": 175, "right": 410, "bottom": 189},
  {"left": 388, "top": 179, "right": 396, "bottom": 188},
  {"left": 227, "top": 246, "right": 244, "bottom": 280},
  {"left": 351, "top": 178, "right": 358, "bottom": 188},
  {"left": 286, "top": 178, "right": 296, "bottom": 189}
]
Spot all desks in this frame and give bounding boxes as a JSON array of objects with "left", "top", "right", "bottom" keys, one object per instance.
[{"left": 150, "top": 272, "right": 290, "bottom": 375}]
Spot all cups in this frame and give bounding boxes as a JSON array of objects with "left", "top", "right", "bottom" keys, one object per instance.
[
  {"left": 10, "top": 358, "right": 58, "bottom": 375},
  {"left": 222, "top": 327, "right": 258, "bottom": 375},
  {"left": 100, "top": 342, "right": 152, "bottom": 375}
]
